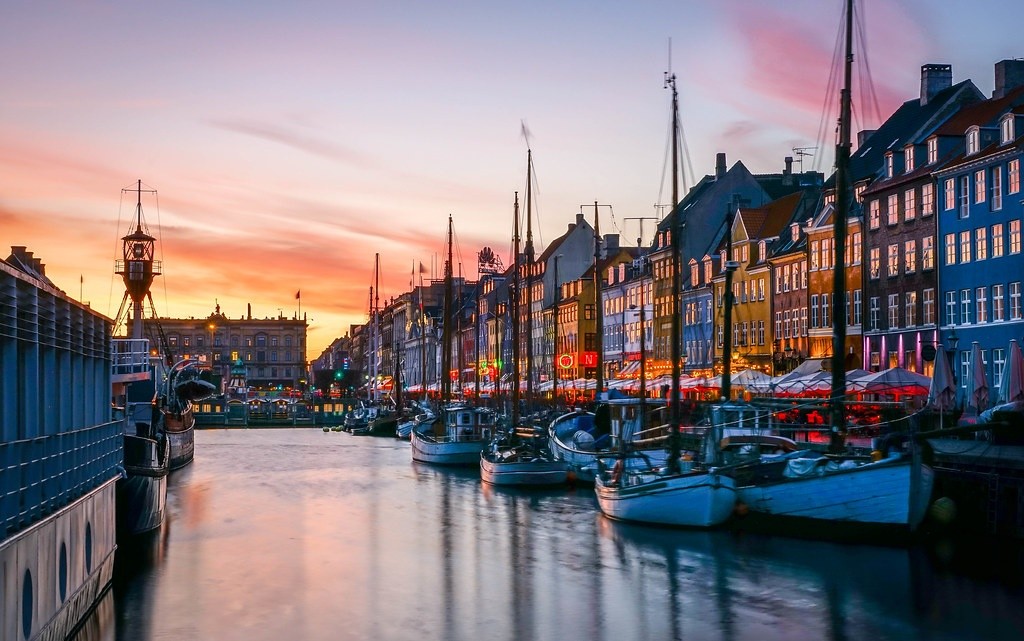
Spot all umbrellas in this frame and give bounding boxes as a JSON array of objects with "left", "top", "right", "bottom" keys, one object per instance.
[
  {"left": 998, "top": 338, "right": 1024, "bottom": 407},
  {"left": 965, "top": 341, "right": 990, "bottom": 426},
  {"left": 929, "top": 344, "right": 956, "bottom": 431}
]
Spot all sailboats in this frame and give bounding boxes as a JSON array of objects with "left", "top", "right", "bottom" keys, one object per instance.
[{"left": 344, "top": 0, "right": 936, "bottom": 532}]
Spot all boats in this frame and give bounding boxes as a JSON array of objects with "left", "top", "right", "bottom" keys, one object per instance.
[
  {"left": 97, "top": 179, "right": 215, "bottom": 536},
  {"left": 1, "top": 246, "right": 127, "bottom": 641}
]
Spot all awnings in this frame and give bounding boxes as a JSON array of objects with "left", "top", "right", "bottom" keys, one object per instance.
[
  {"left": 245, "top": 396, "right": 292, "bottom": 405},
  {"left": 620, "top": 361, "right": 641, "bottom": 374},
  {"left": 400, "top": 363, "right": 931, "bottom": 417},
  {"left": 364, "top": 376, "right": 391, "bottom": 387}
]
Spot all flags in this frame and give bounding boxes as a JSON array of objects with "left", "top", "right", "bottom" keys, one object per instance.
[{"left": 296, "top": 291, "right": 300, "bottom": 299}]
